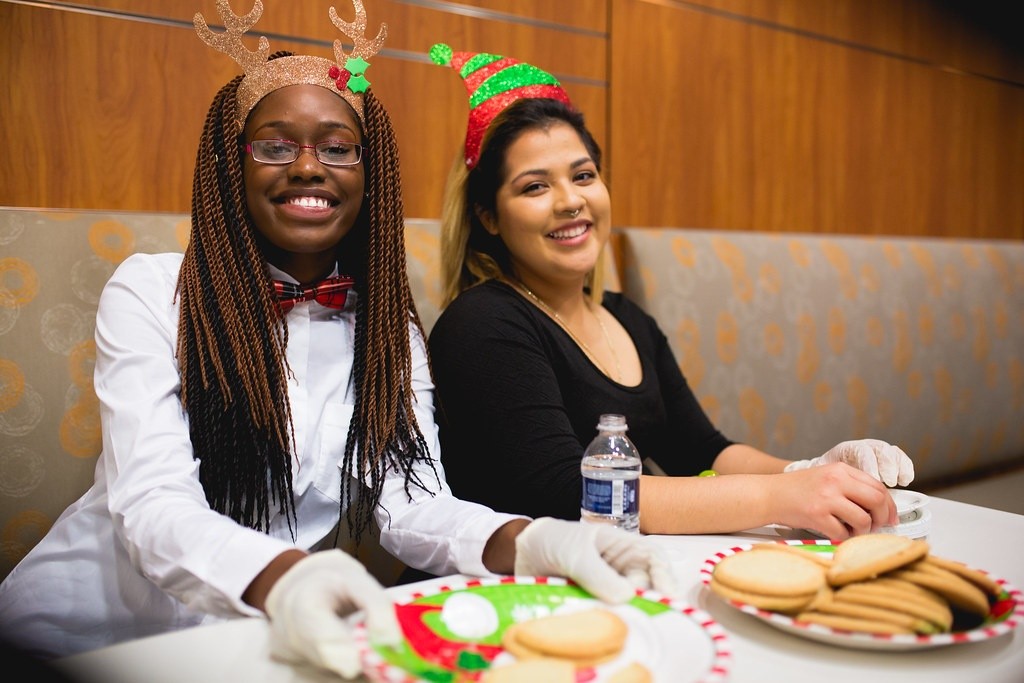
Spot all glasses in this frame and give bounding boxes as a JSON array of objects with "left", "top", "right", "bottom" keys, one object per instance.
[{"left": 236, "top": 136, "right": 368, "bottom": 166}]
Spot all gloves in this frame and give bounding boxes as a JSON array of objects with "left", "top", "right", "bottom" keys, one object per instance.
[
  {"left": 785, "top": 437, "right": 915, "bottom": 490},
  {"left": 511, "top": 514, "right": 675, "bottom": 606},
  {"left": 265, "top": 547, "right": 402, "bottom": 680}
]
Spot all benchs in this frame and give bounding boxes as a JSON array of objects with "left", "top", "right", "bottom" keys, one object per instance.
[
  {"left": 0, "top": 208, "right": 617, "bottom": 586},
  {"left": 623, "top": 224, "right": 1022, "bottom": 513}
]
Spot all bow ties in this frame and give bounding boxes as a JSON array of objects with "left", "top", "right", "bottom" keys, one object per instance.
[{"left": 267, "top": 276, "right": 353, "bottom": 323}]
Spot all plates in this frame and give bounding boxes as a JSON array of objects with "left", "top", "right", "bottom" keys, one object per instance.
[
  {"left": 700, "top": 540, "right": 1023, "bottom": 652},
  {"left": 353, "top": 577, "right": 731, "bottom": 683},
  {"left": 886, "top": 489, "right": 930, "bottom": 516}
]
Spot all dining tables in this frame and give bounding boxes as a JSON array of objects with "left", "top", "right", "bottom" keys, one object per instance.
[{"left": 49, "top": 470, "right": 1022, "bottom": 683}]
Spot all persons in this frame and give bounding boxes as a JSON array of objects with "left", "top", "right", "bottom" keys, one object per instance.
[
  {"left": 424, "top": 96, "right": 916, "bottom": 545},
  {"left": 3, "top": 51, "right": 676, "bottom": 679}
]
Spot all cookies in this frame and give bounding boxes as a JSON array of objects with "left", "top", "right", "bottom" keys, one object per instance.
[{"left": 477, "top": 530, "right": 1004, "bottom": 683}]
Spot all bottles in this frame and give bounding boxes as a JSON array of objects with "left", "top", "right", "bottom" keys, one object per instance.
[{"left": 580, "top": 414, "right": 642, "bottom": 537}]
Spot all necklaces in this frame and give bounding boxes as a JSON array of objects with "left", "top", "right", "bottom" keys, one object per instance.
[{"left": 508, "top": 276, "right": 623, "bottom": 381}]
